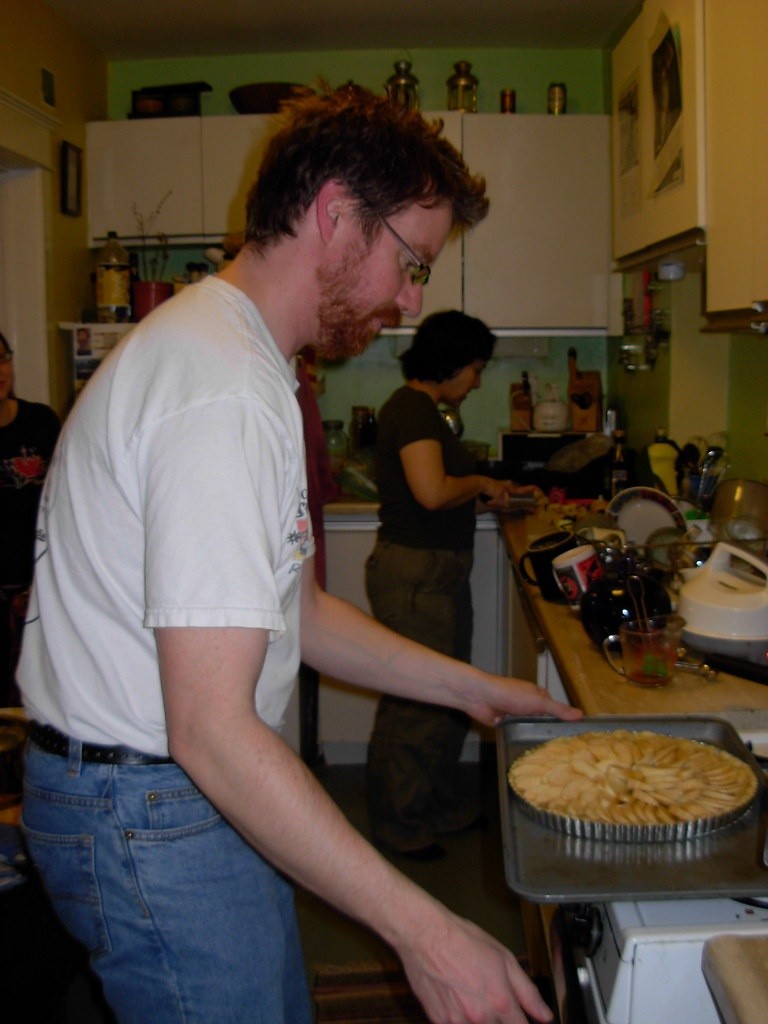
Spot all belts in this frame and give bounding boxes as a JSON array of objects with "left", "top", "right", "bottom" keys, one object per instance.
[{"left": 26, "top": 719, "right": 175, "bottom": 764}]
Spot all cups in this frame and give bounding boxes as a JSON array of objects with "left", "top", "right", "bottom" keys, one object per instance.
[
  {"left": 602, "top": 615, "right": 687, "bottom": 689},
  {"left": 519, "top": 530, "right": 578, "bottom": 605},
  {"left": 551, "top": 545, "right": 603, "bottom": 617},
  {"left": 602, "top": 408, "right": 616, "bottom": 431},
  {"left": 500, "top": 88, "right": 515, "bottom": 114}
]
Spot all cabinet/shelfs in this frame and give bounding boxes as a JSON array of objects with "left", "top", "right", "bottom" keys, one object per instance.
[
  {"left": 603, "top": 0, "right": 768, "bottom": 335},
  {"left": 318, "top": 514, "right": 507, "bottom": 766},
  {"left": 84, "top": 114, "right": 284, "bottom": 249},
  {"left": 399, "top": 110, "right": 612, "bottom": 329},
  {"left": 505, "top": 562, "right": 568, "bottom": 717}
]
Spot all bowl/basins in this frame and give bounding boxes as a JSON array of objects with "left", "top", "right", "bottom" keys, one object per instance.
[{"left": 229, "top": 82, "right": 315, "bottom": 116}]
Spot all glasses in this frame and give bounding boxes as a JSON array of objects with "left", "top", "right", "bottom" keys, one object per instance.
[
  {"left": 0, "top": 351, "right": 14, "bottom": 364},
  {"left": 347, "top": 177, "right": 430, "bottom": 289}
]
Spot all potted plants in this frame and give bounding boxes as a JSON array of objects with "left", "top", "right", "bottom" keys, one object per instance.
[{"left": 130, "top": 188, "right": 175, "bottom": 323}]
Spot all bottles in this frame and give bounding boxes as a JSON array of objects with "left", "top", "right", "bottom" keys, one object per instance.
[
  {"left": 441, "top": 405, "right": 464, "bottom": 441},
  {"left": 647, "top": 427, "right": 681, "bottom": 496},
  {"left": 385, "top": 60, "right": 420, "bottom": 111},
  {"left": 609, "top": 429, "right": 636, "bottom": 500},
  {"left": 96, "top": 231, "right": 130, "bottom": 323},
  {"left": 350, "top": 406, "right": 377, "bottom": 460},
  {"left": 447, "top": 60, "right": 478, "bottom": 114},
  {"left": 322, "top": 420, "right": 349, "bottom": 470},
  {"left": 532, "top": 400, "right": 571, "bottom": 432}
]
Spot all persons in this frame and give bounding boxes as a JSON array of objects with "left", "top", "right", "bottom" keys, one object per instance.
[
  {"left": 366, "top": 310, "right": 515, "bottom": 857},
  {"left": 0, "top": 333, "right": 64, "bottom": 799},
  {"left": 14, "top": 80, "right": 583, "bottom": 1024}
]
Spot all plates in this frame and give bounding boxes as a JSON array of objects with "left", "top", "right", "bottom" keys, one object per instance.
[{"left": 602, "top": 486, "right": 687, "bottom": 546}]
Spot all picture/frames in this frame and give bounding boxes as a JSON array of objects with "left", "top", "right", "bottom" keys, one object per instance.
[{"left": 58, "top": 140, "right": 82, "bottom": 218}]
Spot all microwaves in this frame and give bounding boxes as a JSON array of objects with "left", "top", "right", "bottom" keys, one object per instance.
[{"left": 497, "top": 430, "right": 602, "bottom": 479}]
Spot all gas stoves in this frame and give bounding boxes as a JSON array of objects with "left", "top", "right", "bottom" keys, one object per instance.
[{"left": 542, "top": 728, "right": 768, "bottom": 1024}]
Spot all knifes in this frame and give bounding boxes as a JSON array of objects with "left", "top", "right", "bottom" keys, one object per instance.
[{"left": 479, "top": 492, "right": 536, "bottom": 506}]
